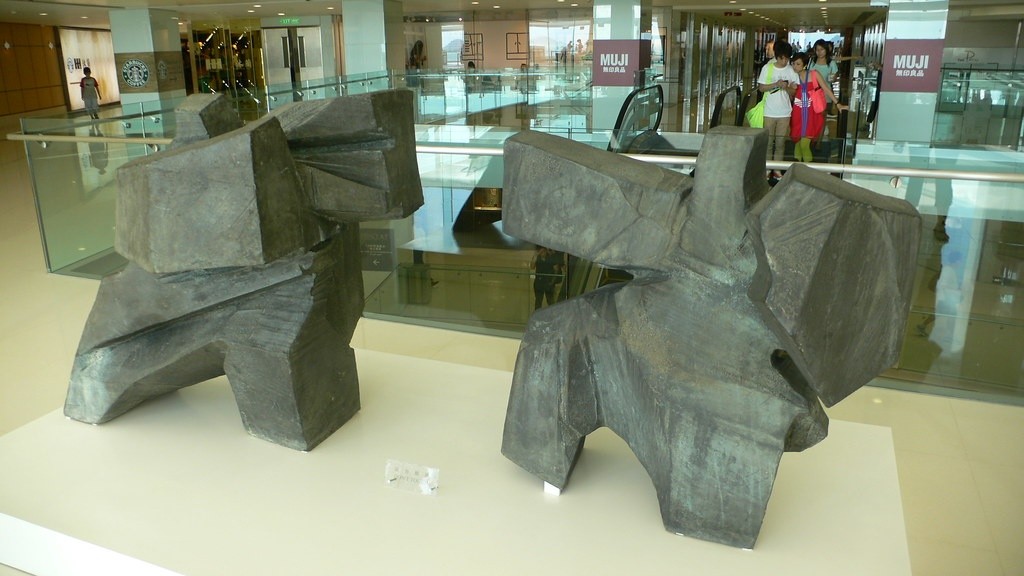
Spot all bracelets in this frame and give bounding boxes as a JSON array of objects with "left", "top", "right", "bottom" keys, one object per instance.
[{"left": 835, "top": 101, "right": 840, "bottom": 105}]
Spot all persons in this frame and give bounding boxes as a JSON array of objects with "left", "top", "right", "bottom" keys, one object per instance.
[
  {"left": 466, "top": 61, "right": 480, "bottom": 82},
  {"left": 917, "top": 248, "right": 964, "bottom": 341},
  {"left": 79, "top": 67, "right": 102, "bottom": 119},
  {"left": 407, "top": 40, "right": 427, "bottom": 85},
  {"left": 757, "top": 39, "right": 850, "bottom": 180},
  {"left": 529, "top": 245, "right": 561, "bottom": 311}
]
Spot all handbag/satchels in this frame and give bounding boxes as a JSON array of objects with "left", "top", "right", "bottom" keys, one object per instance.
[
  {"left": 808, "top": 71, "right": 827, "bottom": 113},
  {"left": 745, "top": 99, "right": 765, "bottom": 129}
]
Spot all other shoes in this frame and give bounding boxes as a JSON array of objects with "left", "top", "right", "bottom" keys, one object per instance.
[
  {"left": 766, "top": 170, "right": 771, "bottom": 180},
  {"left": 772, "top": 172, "right": 782, "bottom": 181},
  {"left": 92, "top": 114, "right": 99, "bottom": 119}
]
